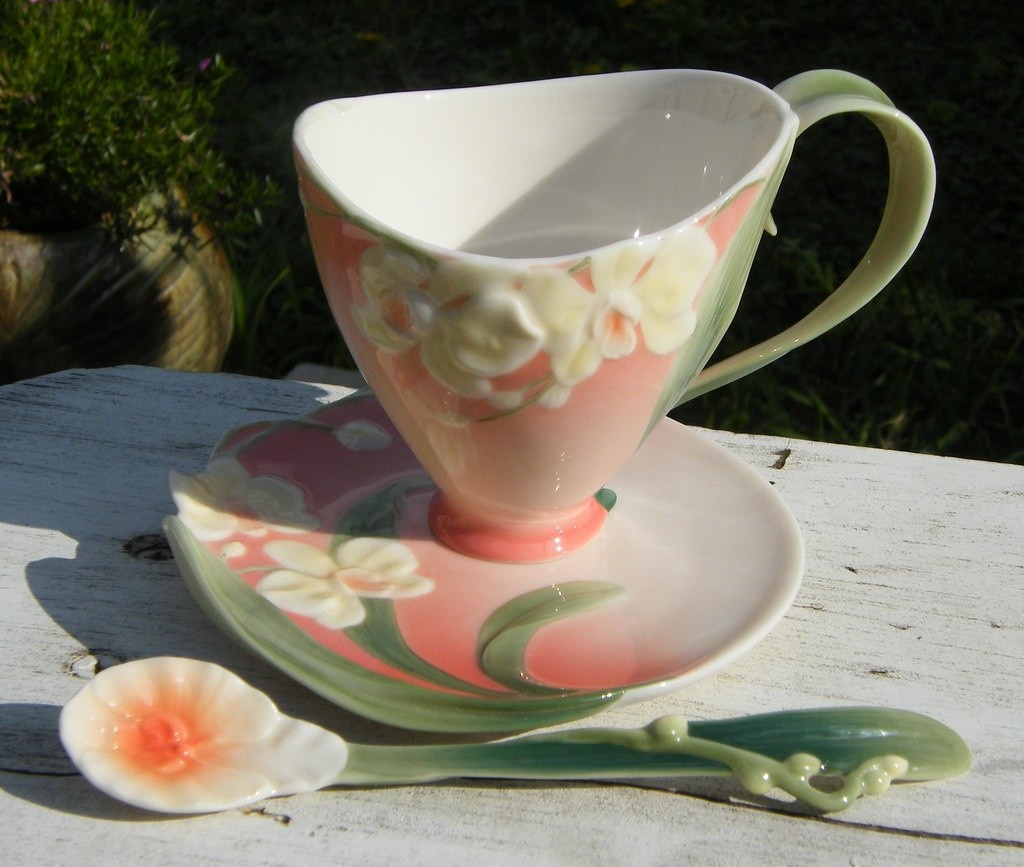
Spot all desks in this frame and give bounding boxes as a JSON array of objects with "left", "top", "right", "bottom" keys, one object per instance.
[{"left": 0, "top": 364, "right": 1024, "bottom": 867}]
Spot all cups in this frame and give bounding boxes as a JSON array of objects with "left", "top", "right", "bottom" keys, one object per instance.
[{"left": 287, "top": 65, "right": 937, "bottom": 564}]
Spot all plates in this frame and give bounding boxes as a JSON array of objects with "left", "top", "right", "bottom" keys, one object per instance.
[{"left": 161, "top": 391, "right": 804, "bottom": 737}]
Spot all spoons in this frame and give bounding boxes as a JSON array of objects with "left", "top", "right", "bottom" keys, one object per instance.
[{"left": 55, "top": 655, "right": 967, "bottom": 816}]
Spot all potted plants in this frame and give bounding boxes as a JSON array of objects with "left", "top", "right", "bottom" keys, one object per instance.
[{"left": 0, "top": 0, "right": 239, "bottom": 377}]
